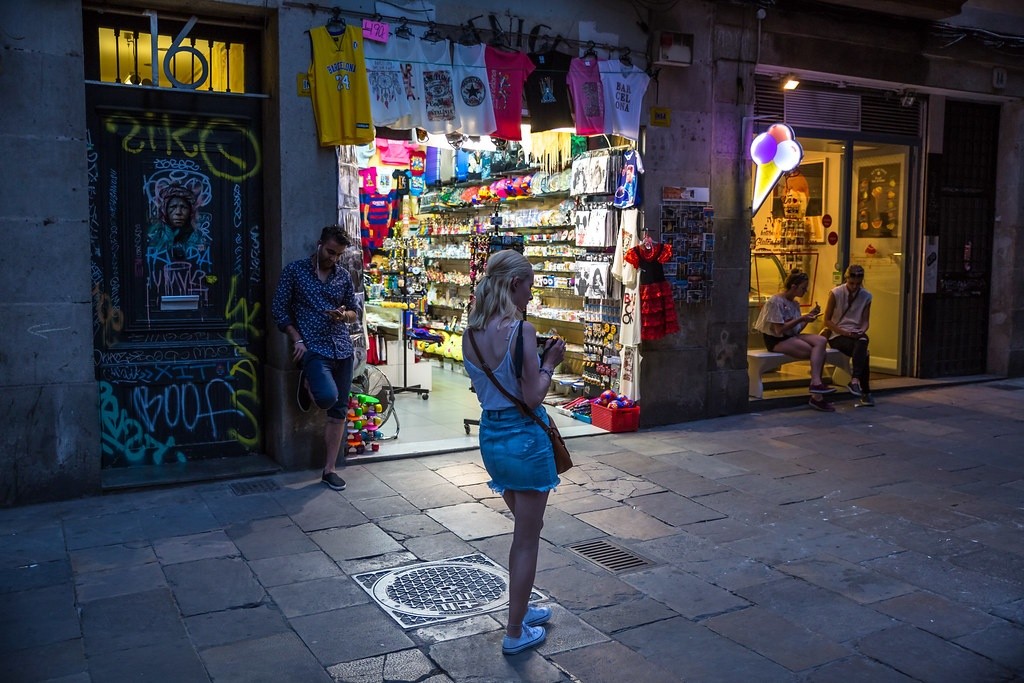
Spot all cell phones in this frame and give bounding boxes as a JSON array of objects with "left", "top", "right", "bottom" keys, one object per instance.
[
  {"left": 325, "top": 310, "right": 344, "bottom": 319},
  {"left": 813, "top": 313, "right": 824, "bottom": 318}
]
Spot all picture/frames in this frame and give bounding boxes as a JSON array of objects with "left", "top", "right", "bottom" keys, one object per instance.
[{"left": 770, "top": 157, "right": 828, "bottom": 244}]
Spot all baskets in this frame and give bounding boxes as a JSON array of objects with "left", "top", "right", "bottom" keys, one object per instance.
[{"left": 590, "top": 397, "right": 640, "bottom": 433}]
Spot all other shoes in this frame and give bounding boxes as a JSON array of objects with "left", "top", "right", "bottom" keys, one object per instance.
[
  {"left": 296, "top": 370, "right": 313, "bottom": 413},
  {"left": 809, "top": 396, "right": 835, "bottom": 412},
  {"left": 809, "top": 384, "right": 837, "bottom": 394},
  {"left": 859, "top": 393, "right": 875, "bottom": 407},
  {"left": 846, "top": 381, "right": 862, "bottom": 396},
  {"left": 321, "top": 469, "right": 346, "bottom": 490}
]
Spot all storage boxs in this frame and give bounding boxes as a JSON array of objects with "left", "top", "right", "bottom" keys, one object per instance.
[
  {"left": 591, "top": 399, "right": 641, "bottom": 432},
  {"left": 554, "top": 382, "right": 569, "bottom": 395},
  {"left": 569, "top": 383, "right": 583, "bottom": 397}
]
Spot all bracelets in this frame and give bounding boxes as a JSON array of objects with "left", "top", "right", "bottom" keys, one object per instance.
[
  {"left": 293, "top": 339, "right": 304, "bottom": 346},
  {"left": 344, "top": 314, "right": 349, "bottom": 325},
  {"left": 539, "top": 368, "right": 553, "bottom": 383}
]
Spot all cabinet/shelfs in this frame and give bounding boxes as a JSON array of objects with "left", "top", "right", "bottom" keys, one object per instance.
[{"left": 417, "top": 144, "right": 627, "bottom": 380}]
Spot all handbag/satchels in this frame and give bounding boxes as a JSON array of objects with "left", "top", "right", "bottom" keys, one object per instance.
[
  {"left": 818, "top": 326, "right": 833, "bottom": 342},
  {"left": 546, "top": 413, "right": 574, "bottom": 475}
]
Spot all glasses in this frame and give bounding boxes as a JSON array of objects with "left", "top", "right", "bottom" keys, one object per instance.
[{"left": 844, "top": 271, "right": 865, "bottom": 280}]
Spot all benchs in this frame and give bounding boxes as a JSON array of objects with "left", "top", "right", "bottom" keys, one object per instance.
[{"left": 748, "top": 345, "right": 870, "bottom": 397}]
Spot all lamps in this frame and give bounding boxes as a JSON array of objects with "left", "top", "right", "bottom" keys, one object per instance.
[
  {"left": 124, "top": 32, "right": 142, "bottom": 84},
  {"left": 897, "top": 89, "right": 916, "bottom": 107},
  {"left": 777, "top": 72, "right": 801, "bottom": 90}
]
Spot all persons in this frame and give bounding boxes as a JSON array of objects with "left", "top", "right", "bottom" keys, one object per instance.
[
  {"left": 825, "top": 265, "right": 876, "bottom": 406},
  {"left": 755, "top": 267, "right": 837, "bottom": 412},
  {"left": 461, "top": 248, "right": 566, "bottom": 656},
  {"left": 273, "top": 224, "right": 362, "bottom": 491}
]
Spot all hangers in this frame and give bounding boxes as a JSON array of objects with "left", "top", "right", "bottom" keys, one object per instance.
[
  {"left": 418, "top": 20, "right": 447, "bottom": 42},
  {"left": 451, "top": 25, "right": 489, "bottom": 46},
  {"left": 619, "top": 47, "right": 634, "bottom": 67},
  {"left": 490, "top": 33, "right": 520, "bottom": 51},
  {"left": 641, "top": 227, "right": 658, "bottom": 247},
  {"left": 393, "top": 14, "right": 417, "bottom": 38},
  {"left": 372, "top": 13, "right": 392, "bottom": 37},
  {"left": 531, "top": 36, "right": 553, "bottom": 53},
  {"left": 305, "top": 7, "right": 368, "bottom": 33},
  {"left": 578, "top": 40, "right": 598, "bottom": 61}
]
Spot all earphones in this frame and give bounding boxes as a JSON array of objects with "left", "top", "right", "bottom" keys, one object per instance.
[{"left": 318, "top": 244, "right": 322, "bottom": 251}]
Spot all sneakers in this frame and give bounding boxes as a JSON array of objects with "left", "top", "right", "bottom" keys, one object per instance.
[
  {"left": 502, "top": 620, "right": 546, "bottom": 655},
  {"left": 523, "top": 606, "right": 552, "bottom": 627}
]
